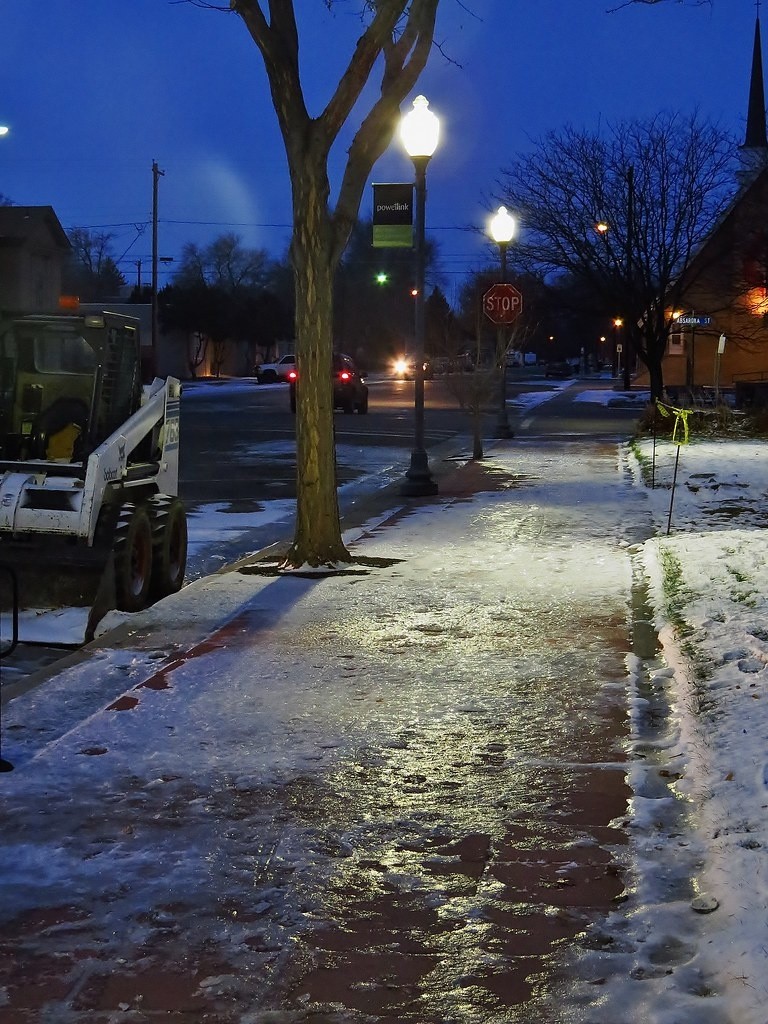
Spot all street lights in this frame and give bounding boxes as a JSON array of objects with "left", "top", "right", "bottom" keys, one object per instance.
[
  {"left": 398, "top": 94, "right": 441, "bottom": 497},
  {"left": 485, "top": 207, "right": 517, "bottom": 440}
]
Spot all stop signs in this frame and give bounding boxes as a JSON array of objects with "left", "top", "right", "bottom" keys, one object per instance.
[{"left": 485, "top": 284, "right": 522, "bottom": 327}]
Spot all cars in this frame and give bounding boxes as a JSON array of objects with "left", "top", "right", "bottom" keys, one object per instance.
[
  {"left": 289, "top": 351, "right": 370, "bottom": 415},
  {"left": 391, "top": 354, "right": 475, "bottom": 380}
]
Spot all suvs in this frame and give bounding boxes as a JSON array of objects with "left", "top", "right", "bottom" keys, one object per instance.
[{"left": 257, "top": 353, "right": 297, "bottom": 384}]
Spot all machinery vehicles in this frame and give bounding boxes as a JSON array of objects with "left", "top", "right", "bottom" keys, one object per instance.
[{"left": 0, "top": 291, "right": 186, "bottom": 646}]
